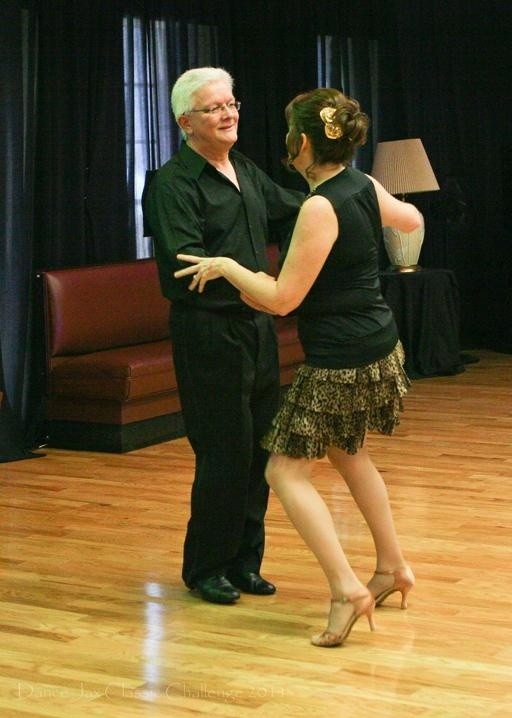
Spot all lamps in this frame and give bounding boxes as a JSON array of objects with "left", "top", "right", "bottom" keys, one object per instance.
[{"left": 370, "top": 136, "right": 442, "bottom": 274}]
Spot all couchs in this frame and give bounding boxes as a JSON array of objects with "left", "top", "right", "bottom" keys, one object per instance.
[{"left": 26, "top": 239, "right": 304, "bottom": 456}]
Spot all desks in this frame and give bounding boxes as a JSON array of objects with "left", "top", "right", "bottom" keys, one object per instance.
[{"left": 372, "top": 263, "right": 466, "bottom": 367}]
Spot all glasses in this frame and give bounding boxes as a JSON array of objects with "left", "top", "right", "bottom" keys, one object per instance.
[{"left": 183, "top": 101, "right": 242, "bottom": 115}]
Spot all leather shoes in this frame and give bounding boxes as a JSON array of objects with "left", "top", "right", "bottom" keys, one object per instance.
[
  {"left": 184, "top": 573, "right": 240, "bottom": 602},
  {"left": 229, "top": 570, "right": 276, "bottom": 595}
]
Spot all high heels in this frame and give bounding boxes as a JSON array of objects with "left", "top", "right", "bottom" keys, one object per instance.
[
  {"left": 309, "top": 591, "right": 375, "bottom": 647},
  {"left": 375, "top": 566, "right": 416, "bottom": 609}
]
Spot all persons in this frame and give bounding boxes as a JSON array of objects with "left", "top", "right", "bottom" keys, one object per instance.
[{"left": 146, "top": 60, "right": 424, "bottom": 646}]
